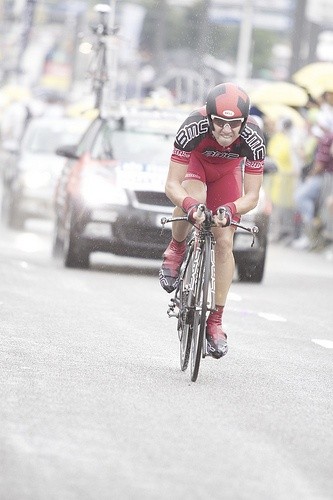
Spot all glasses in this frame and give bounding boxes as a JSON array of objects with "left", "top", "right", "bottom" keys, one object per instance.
[{"left": 210, "top": 117, "right": 242, "bottom": 128}]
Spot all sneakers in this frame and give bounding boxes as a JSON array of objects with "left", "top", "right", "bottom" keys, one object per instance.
[
  {"left": 158, "top": 246, "right": 187, "bottom": 293},
  {"left": 205, "top": 318, "right": 229, "bottom": 359}
]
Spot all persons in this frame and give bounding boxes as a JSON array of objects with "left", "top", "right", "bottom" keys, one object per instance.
[
  {"left": 249, "top": 89, "right": 333, "bottom": 254},
  {"left": 157, "top": 82, "right": 265, "bottom": 360}
]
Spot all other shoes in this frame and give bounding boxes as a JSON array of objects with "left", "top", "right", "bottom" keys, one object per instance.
[{"left": 272, "top": 228, "right": 332, "bottom": 251}]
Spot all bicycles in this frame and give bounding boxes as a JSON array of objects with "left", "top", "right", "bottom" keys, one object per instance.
[{"left": 161, "top": 205, "right": 259, "bottom": 382}]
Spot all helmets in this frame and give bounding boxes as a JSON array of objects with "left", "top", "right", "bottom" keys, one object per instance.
[{"left": 205, "top": 82, "right": 251, "bottom": 135}]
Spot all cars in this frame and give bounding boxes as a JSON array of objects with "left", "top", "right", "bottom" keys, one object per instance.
[
  {"left": 54, "top": 111, "right": 268, "bottom": 279},
  {"left": 2, "top": 114, "right": 87, "bottom": 231}
]
